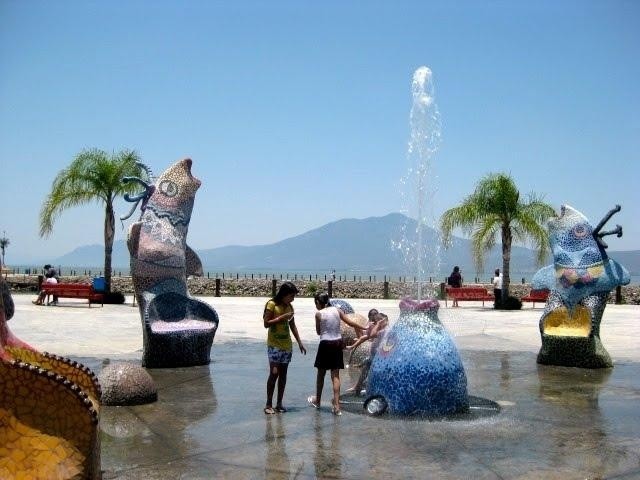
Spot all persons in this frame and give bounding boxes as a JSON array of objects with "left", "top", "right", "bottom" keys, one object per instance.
[
  {"left": 492, "top": 269, "right": 503, "bottom": 309},
  {"left": 259, "top": 281, "right": 306, "bottom": 414},
  {"left": 450, "top": 266, "right": 462, "bottom": 306},
  {"left": 44, "top": 264, "right": 59, "bottom": 305},
  {"left": 345, "top": 313, "right": 390, "bottom": 393},
  {"left": 306, "top": 291, "right": 374, "bottom": 416},
  {"left": 28, "top": 273, "right": 57, "bottom": 305},
  {"left": 344, "top": 307, "right": 378, "bottom": 351}
]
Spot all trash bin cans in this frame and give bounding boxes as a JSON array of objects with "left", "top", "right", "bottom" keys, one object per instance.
[{"left": 93, "top": 277, "right": 105, "bottom": 290}]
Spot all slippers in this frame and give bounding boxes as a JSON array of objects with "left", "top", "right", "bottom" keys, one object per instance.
[
  {"left": 263, "top": 407, "right": 275, "bottom": 414},
  {"left": 276, "top": 407, "right": 286, "bottom": 412},
  {"left": 308, "top": 395, "right": 320, "bottom": 409},
  {"left": 331, "top": 406, "right": 342, "bottom": 416}
]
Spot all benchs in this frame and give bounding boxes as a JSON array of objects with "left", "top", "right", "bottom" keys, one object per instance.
[
  {"left": 39, "top": 280, "right": 105, "bottom": 309},
  {"left": 443, "top": 286, "right": 496, "bottom": 309},
  {"left": 521, "top": 287, "right": 552, "bottom": 309}
]
